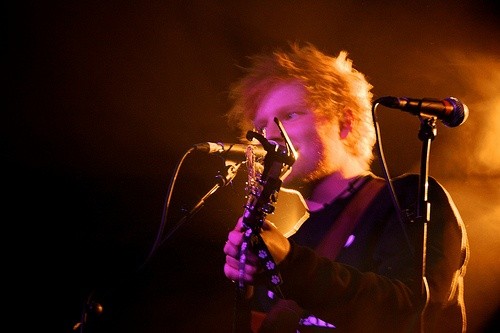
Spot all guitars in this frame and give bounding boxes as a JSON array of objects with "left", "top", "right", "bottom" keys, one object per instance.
[{"left": 229, "top": 117, "right": 299, "bottom": 333}]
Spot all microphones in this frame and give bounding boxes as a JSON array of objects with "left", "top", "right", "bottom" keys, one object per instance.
[
  {"left": 192, "top": 142, "right": 289, "bottom": 166},
  {"left": 376, "top": 95, "right": 472, "bottom": 128}
]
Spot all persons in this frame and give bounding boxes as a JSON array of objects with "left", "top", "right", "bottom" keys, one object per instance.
[{"left": 219, "top": 44, "right": 471, "bottom": 332}]
